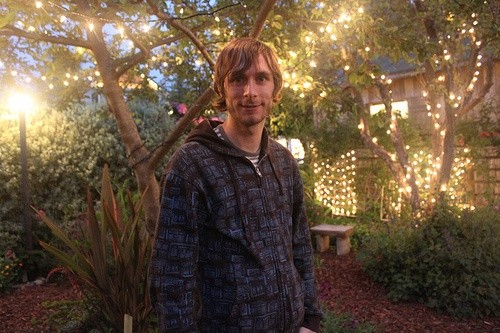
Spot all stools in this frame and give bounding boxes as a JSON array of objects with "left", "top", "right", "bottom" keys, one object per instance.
[{"left": 310, "top": 223, "right": 354, "bottom": 256}]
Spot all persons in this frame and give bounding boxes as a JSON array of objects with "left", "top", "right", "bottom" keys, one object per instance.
[{"left": 148, "top": 39, "right": 325, "bottom": 333}]
